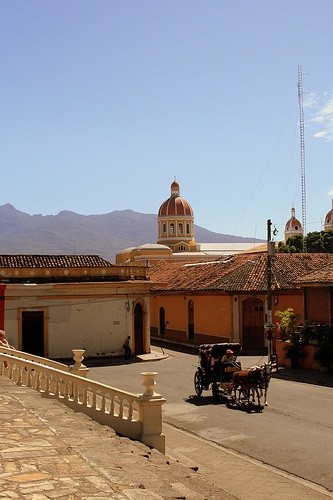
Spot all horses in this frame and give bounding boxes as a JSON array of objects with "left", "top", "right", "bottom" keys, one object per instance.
[{"left": 233, "top": 361, "right": 271, "bottom": 406}]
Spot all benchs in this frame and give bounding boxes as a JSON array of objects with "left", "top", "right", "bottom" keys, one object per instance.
[{"left": 210, "top": 362, "right": 223, "bottom": 378}]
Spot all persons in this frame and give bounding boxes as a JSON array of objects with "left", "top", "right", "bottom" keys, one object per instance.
[
  {"left": 208, "top": 354, "right": 218, "bottom": 365},
  {"left": 0, "top": 330, "right": 15, "bottom": 349},
  {"left": 221, "top": 349, "right": 235, "bottom": 363},
  {"left": 124, "top": 336, "right": 131, "bottom": 359}
]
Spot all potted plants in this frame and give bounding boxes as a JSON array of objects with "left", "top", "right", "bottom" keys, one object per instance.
[
  {"left": 299, "top": 320, "right": 315, "bottom": 345},
  {"left": 313, "top": 326, "right": 333, "bottom": 370},
  {"left": 282, "top": 342, "right": 308, "bottom": 368},
  {"left": 274, "top": 307, "right": 300, "bottom": 343}
]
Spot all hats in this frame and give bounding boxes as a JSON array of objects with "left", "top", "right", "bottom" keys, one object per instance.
[{"left": 226, "top": 349, "right": 234, "bottom": 354}]
